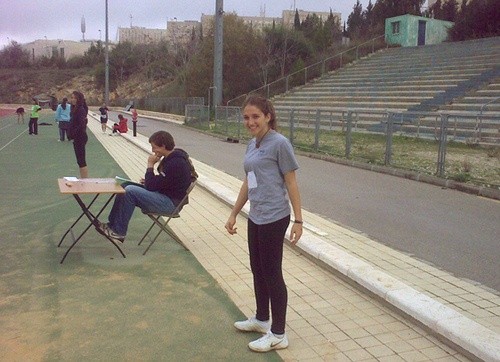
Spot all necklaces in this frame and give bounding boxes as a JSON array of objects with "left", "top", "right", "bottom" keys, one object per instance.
[{"left": 256, "top": 129, "right": 270, "bottom": 148}]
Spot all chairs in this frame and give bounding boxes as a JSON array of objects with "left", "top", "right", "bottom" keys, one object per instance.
[{"left": 138, "top": 174, "right": 199, "bottom": 257}]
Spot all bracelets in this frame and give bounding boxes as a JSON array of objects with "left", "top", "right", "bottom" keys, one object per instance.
[{"left": 295, "top": 220, "right": 303, "bottom": 224}]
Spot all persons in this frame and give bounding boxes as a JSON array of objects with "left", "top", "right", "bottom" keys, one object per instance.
[
  {"left": 15, "top": 97, "right": 71, "bottom": 142},
  {"left": 67, "top": 91, "right": 88, "bottom": 179},
  {"left": 99, "top": 102, "right": 129, "bottom": 136},
  {"left": 225, "top": 94, "right": 304, "bottom": 352},
  {"left": 95, "top": 131, "right": 191, "bottom": 244}
]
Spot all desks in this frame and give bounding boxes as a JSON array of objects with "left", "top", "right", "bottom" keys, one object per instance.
[{"left": 57, "top": 177, "right": 127, "bottom": 264}]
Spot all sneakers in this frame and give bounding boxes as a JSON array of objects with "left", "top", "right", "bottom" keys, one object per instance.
[
  {"left": 248, "top": 331, "right": 288, "bottom": 351},
  {"left": 234, "top": 319, "right": 270, "bottom": 333},
  {"left": 99, "top": 223, "right": 125, "bottom": 244}
]
